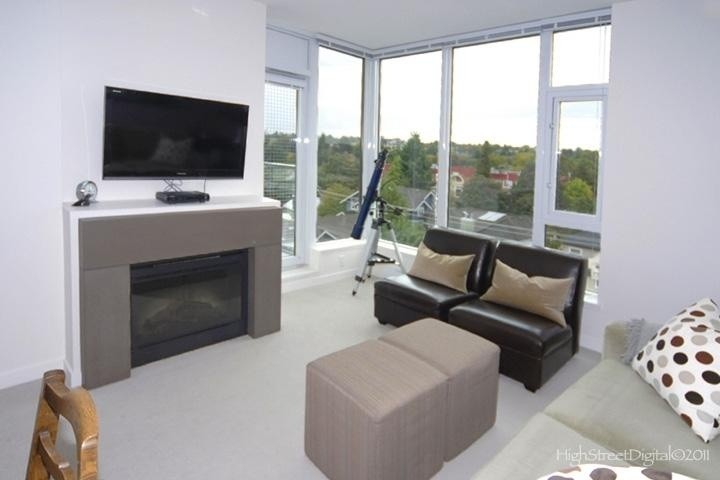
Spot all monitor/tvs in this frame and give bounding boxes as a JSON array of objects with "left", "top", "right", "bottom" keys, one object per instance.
[{"left": 102, "top": 85, "right": 249, "bottom": 181}]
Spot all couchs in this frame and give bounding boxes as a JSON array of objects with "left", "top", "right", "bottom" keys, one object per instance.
[{"left": 458, "top": 318, "right": 719, "bottom": 480}]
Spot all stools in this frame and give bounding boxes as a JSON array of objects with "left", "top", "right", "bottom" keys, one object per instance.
[
  {"left": 304, "top": 338, "right": 447, "bottom": 478},
  {"left": 382, "top": 316, "right": 502, "bottom": 462}
]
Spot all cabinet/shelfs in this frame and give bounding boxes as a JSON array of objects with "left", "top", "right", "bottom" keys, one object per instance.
[{"left": 62, "top": 198, "right": 282, "bottom": 392}]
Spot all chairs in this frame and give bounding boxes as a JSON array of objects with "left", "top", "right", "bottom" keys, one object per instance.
[
  {"left": 374, "top": 226, "right": 500, "bottom": 326},
  {"left": 449, "top": 237, "right": 589, "bottom": 393},
  {"left": 24, "top": 369, "right": 100, "bottom": 480}
]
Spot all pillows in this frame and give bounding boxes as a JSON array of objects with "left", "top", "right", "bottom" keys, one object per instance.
[
  {"left": 406, "top": 239, "right": 474, "bottom": 296},
  {"left": 481, "top": 257, "right": 575, "bottom": 331},
  {"left": 631, "top": 295, "right": 719, "bottom": 444},
  {"left": 536, "top": 464, "right": 696, "bottom": 480}
]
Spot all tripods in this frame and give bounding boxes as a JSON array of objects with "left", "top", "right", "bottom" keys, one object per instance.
[{"left": 352, "top": 199, "right": 406, "bottom": 297}]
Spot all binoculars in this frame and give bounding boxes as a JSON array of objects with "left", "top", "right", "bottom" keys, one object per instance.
[{"left": 350, "top": 149, "right": 389, "bottom": 240}]
